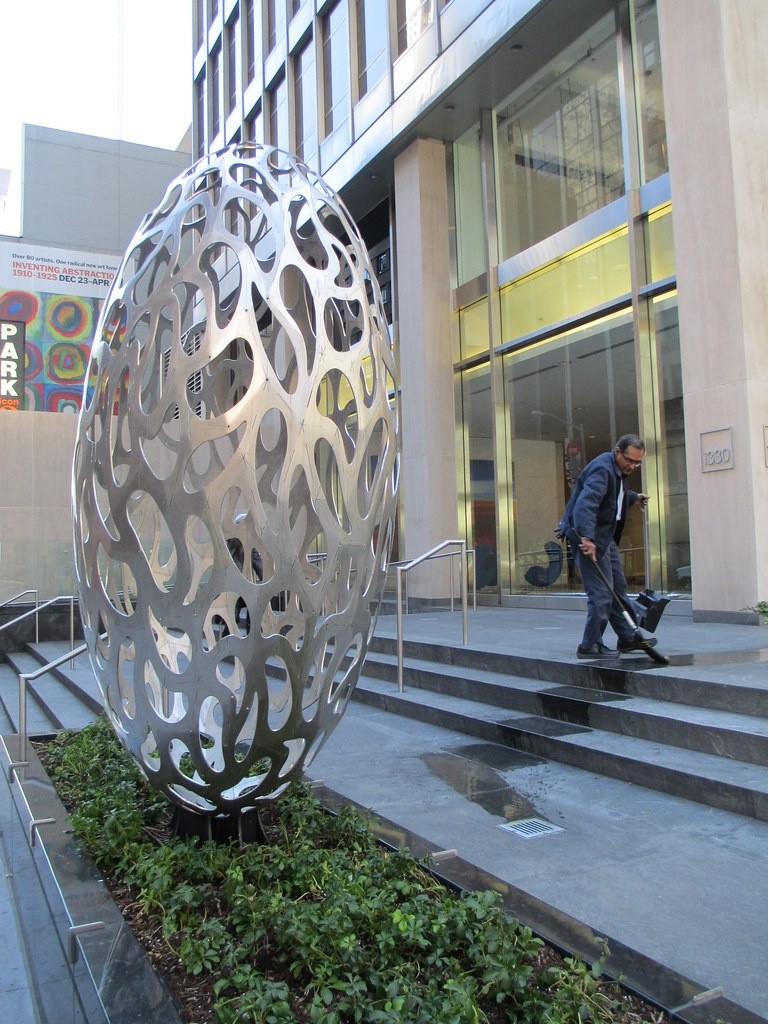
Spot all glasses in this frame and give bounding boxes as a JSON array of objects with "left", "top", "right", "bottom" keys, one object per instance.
[{"left": 621, "top": 452, "right": 642, "bottom": 467}]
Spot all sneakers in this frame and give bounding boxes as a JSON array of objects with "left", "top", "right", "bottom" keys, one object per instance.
[
  {"left": 576, "top": 642, "right": 621, "bottom": 659},
  {"left": 617, "top": 631, "right": 657, "bottom": 653}
]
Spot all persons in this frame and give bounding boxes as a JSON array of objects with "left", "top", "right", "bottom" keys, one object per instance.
[{"left": 556, "top": 435, "right": 658, "bottom": 659}]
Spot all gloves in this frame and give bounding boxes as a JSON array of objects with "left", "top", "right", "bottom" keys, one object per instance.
[{"left": 554, "top": 526, "right": 566, "bottom": 543}]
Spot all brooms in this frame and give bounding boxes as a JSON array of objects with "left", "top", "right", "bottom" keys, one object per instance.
[{"left": 571, "top": 526, "right": 670, "bottom": 665}]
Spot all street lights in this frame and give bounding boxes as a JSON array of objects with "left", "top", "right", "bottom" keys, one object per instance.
[{"left": 530, "top": 410, "right": 589, "bottom": 469}]
